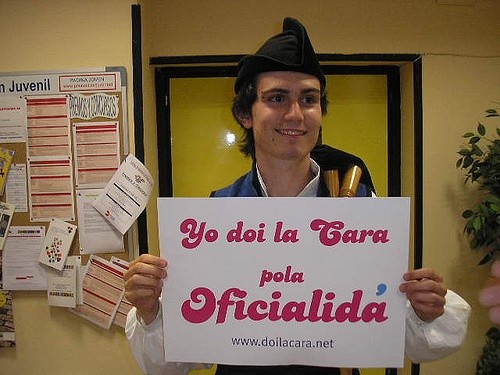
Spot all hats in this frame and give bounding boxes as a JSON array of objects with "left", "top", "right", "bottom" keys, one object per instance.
[{"left": 234, "top": 17, "right": 327, "bottom": 95}]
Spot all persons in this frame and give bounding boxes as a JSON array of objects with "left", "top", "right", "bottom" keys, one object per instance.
[{"left": 122, "top": 29, "right": 472, "bottom": 375}]
